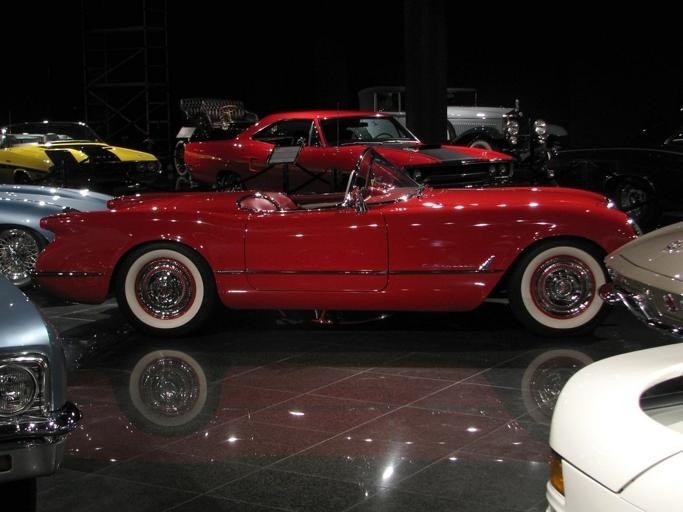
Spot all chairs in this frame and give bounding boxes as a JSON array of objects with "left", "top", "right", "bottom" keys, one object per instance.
[{"left": 242, "top": 193, "right": 298, "bottom": 212}]
